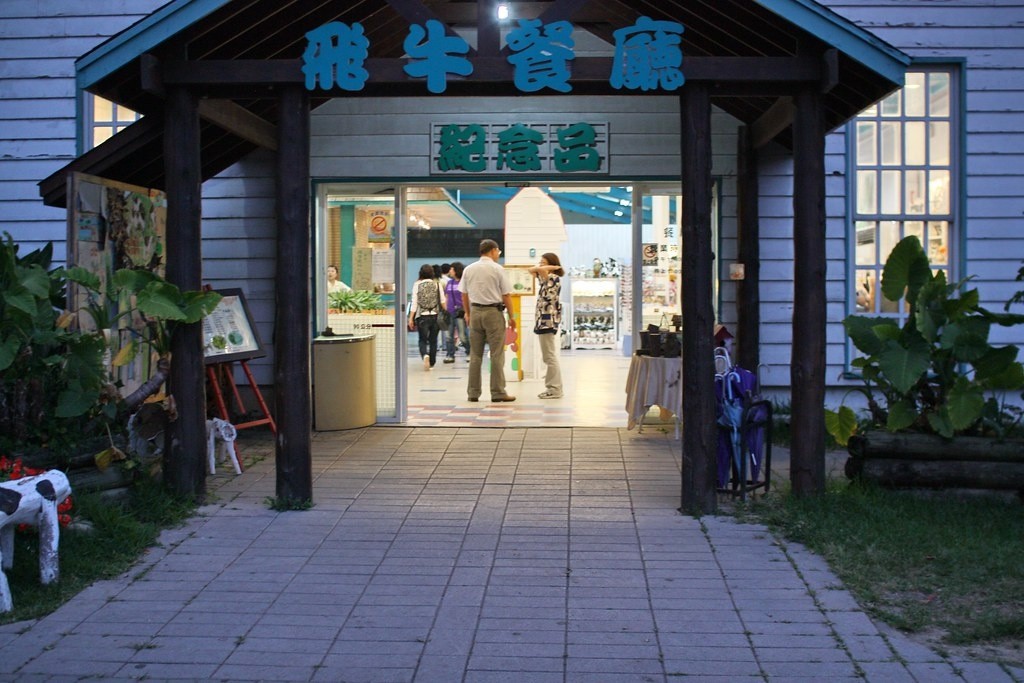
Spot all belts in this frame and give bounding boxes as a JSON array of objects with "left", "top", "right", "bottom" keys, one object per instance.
[{"left": 471, "top": 302, "right": 499, "bottom": 307}]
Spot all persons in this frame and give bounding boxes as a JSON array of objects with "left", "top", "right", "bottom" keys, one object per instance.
[
  {"left": 409, "top": 264, "right": 447, "bottom": 371},
  {"left": 432, "top": 264, "right": 463, "bottom": 350},
  {"left": 456, "top": 239, "right": 516, "bottom": 402},
  {"left": 327, "top": 265, "right": 351, "bottom": 292},
  {"left": 527, "top": 253, "right": 565, "bottom": 399},
  {"left": 443, "top": 262, "right": 470, "bottom": 363}
]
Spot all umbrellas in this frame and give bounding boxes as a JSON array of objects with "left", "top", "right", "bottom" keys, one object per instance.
[{"left": 714, "top": 346, "right": 767, "bottom": 501}]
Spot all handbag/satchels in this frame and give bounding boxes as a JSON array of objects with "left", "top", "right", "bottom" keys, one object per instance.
[
  {"left": 456, "top": 309, "right": 464, "bottom": 318},
  {"left": 437, "top": 310, "right": 450, "bottom": 331}
]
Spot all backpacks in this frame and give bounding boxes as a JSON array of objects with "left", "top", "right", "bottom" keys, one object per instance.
[{"left": 417, "top": 280, "right": 439, "bottom": 312}]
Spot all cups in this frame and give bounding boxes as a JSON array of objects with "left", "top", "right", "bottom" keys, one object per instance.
[
  {"left": 665, "top": 332, "right": 677, "bottom": 357},
  {"left": 649, "top": 334, "right": 661, "bottom": 356},
  {"left": 639, "top": 330, "right": 650, "bottom": 349}
]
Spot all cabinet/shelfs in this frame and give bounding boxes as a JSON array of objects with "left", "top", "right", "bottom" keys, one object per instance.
[{"left": 571, "top": 276, "right": 617, "bottom": 351}]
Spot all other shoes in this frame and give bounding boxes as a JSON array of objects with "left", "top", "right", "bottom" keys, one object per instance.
[
  {"left": 491, "top": 394, "right": 516, "bottom": 402},
  {"left": 423, "top": 354, "right": 430, "bottom": 372},
  {"left": 468, "top": 397, "right": 478, "bottom": 402},
  {"left": 466, "top": 354, "right": 471, "bottom": 362},
  {"left": 430, "top": 367, "right": 434, "bottom": 370},
  {"left": 538, "top": 392, "right": 562, "bottom": 399},
  {"left": 443, "top": 358, "right": 454, "bottom": 364}
]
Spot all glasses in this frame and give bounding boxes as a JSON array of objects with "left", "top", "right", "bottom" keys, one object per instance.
[
  {"left": 539, "top": 262, "right": 549, "bottom": 265},
  {"left": 493, "top": 248, "right": 502, "bottom": 254}
]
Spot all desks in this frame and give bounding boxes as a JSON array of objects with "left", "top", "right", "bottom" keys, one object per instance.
[{"left": 625, "top": 352, "right": 683, "bottom": 440}]
